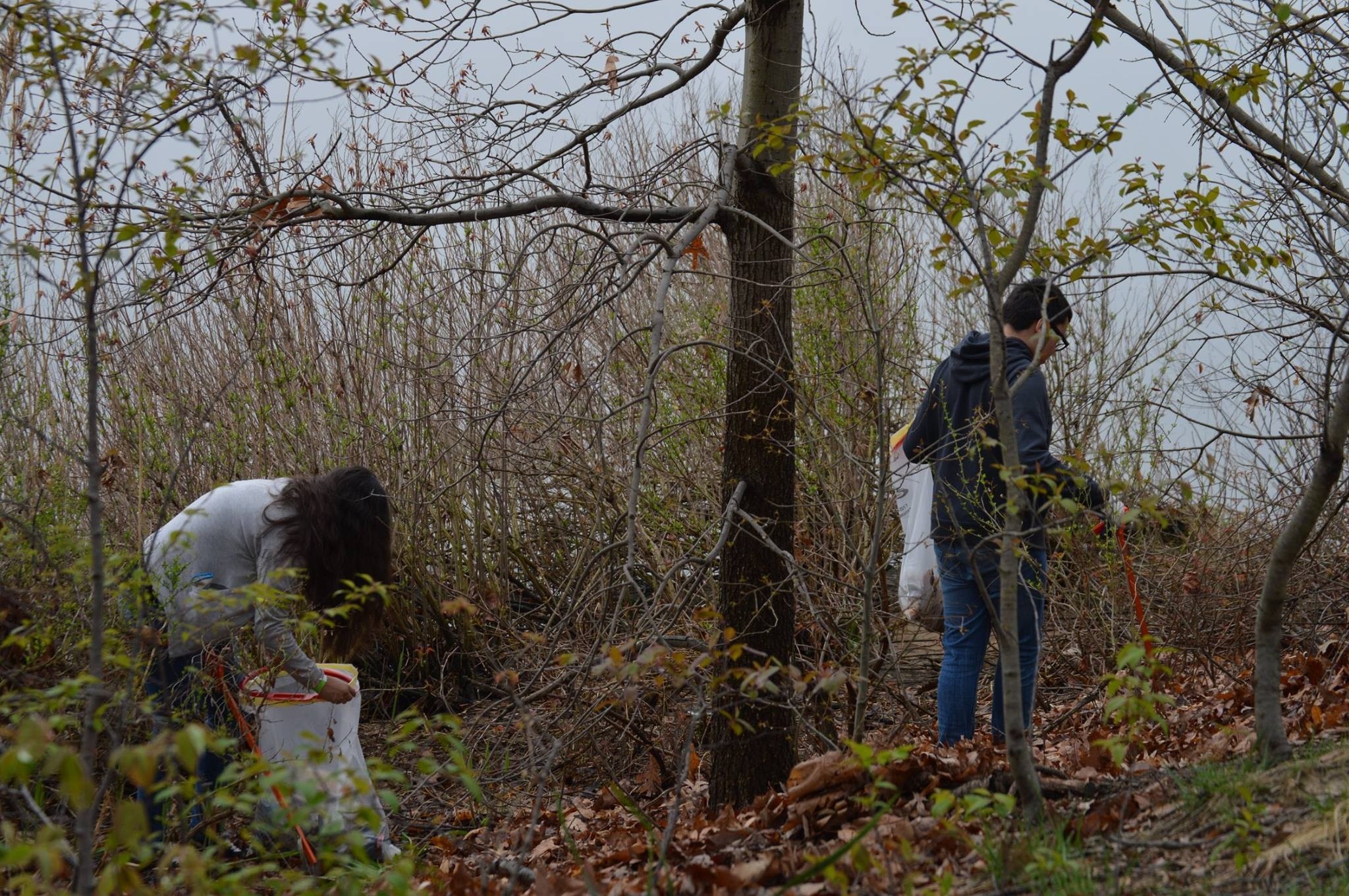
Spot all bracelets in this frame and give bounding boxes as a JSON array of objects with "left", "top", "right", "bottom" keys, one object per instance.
[{"left": 313, "top": 676, "right": 328, "bottom": 692}]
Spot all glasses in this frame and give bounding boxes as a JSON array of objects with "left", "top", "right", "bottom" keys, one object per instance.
[{"left": 1051, "top": 322, "right": 1070, "bottom": 352}]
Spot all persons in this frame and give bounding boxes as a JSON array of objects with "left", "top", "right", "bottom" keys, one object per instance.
[
  {"left": 900, "top": 280, "right": 1109, "bottom": 757},
  {"left": 135, "top": 465, "right": 394, "bottom": 861}
]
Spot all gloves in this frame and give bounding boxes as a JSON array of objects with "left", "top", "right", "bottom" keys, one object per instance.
[{"left": 1100, "top": 490, "right": 1133, "bottom": 527}]
